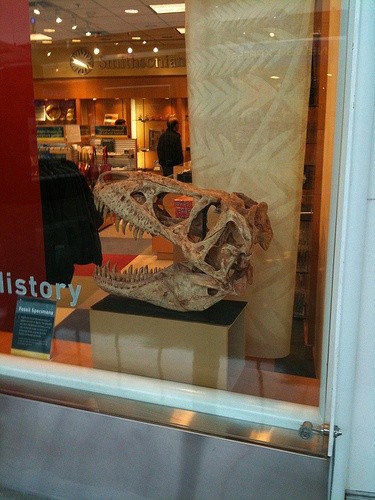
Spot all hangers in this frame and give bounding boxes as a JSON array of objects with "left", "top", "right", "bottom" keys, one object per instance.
[{"left": 38, "top": 143, "right": 68, "bottom": 162}]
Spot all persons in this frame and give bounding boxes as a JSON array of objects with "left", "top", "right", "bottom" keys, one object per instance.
[
  {"left": 114, "top": 119, "right": 128, "bottom": 127},
  {"left": 156, "top": 117, "right": 184, "bottom": 179}
]
